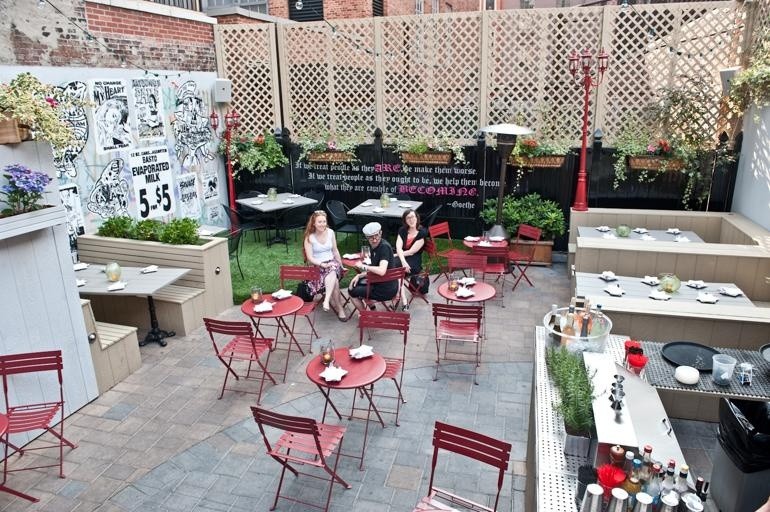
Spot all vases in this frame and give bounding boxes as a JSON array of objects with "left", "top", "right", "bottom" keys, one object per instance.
[
  {"left": 307, "top": 150, "right": 350, "bottom": 163},
  {"left": 627, "top": 154, "right": 686, "bottom": 171},
  {"left": 398, "top": 150, "right": 453, "bottom": 166},
  {"left": 0, "top": 111, "right": 31, "bottom": 144}
]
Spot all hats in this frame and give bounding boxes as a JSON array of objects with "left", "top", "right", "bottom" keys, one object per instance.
[{"left": 363, "top": 222, "right": 381, "bottom": 236}]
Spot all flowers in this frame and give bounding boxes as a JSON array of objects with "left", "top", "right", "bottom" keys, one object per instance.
[
  {"left": 220, "top": 131, "right": 289, "bottom": 179},
  {"left": 0, "top": 71, "right": 97, "bottom": 160},
  {"left": 612, "top": 113, "right": 712, "bottom": 211},
  {"left": 295, "top": 126, "right": 368, "bottom": 166},
  {"left": 393, "top": 133, "right": 470, "bottom": 173}
]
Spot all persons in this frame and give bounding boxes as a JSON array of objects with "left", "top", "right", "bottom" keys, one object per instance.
[
  {"left": 348, "top": 222, "right": 398, "bottom": 312},
  {"left": 390, "top": 209, "right": 426, "bottom": 312},
  {"left": 303, "top": 210, "right": 348, "bottom": 322}
]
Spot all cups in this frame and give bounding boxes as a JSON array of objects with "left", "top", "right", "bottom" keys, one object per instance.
[
  {"left": 447, "top": 273, "right": 458, "bottom": 291},
  {"left": 250, "top": 286, "right": 262, "bottom": 302},
  {"left": 481, "top": 232, "right": 490, "bottom": 243},
  {"left": 319, "top": 342, "right": 335, "bottom": 364},
  {"left": 622, "top": 339, "right": 649, "bottom": 376},
  {"left": 711, "top": 354, "right": 738, "bottom": 387},
  {"left": 361, "top": 246, "right": 370, "bottom": 258}
]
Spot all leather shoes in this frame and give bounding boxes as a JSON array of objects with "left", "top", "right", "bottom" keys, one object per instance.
[
  {"left": 402, "top": 299, "right": 409, "bottom": 311},
  {"left": 337, "top": 312, "right": 347, "bottom": 322},
  {"left": 389, "top": 299, "right": 399, "bottom": 310},
  {"left": 322, "top": 302, "right": 330, "bottom": 312}
]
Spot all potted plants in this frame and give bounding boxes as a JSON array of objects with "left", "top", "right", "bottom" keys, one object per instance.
[
  {"left": 481, "top": 193, "right": 567, "bottom": 266},
  {"left": 546, "top": 345, "right": 606, "bottom": 458}
]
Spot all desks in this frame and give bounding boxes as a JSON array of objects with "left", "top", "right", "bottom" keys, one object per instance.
[
  {"left": 575, "top": 269, "right": 753, "bottom": 306},
  {"left": 578, "top": 224, "right": 704, "bottom": 243}
]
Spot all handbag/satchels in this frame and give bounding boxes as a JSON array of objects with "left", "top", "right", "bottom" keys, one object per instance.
[
  {"left": 297, "top": 283, "right": 314, "bottom": 302},
  {"left": 409, "top": 276, "right": 429, "bottom": 294}
]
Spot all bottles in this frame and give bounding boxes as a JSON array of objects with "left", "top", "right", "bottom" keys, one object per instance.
[
  {"left": 547, "top": 296, "right": 604, "bottom": 349},
  {"left": 576, "top": 445, "right": 711, "bottom": 512},
  {"left": 381, "top": 194, "right": 391, "bottom": 209},
  {"left": 267, "top": 187, "right": 278, "bottom": 202}
]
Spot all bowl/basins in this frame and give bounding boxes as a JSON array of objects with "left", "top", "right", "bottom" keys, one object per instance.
[{"left": 543, "top": 307, "right": 613, "bottom": 352}]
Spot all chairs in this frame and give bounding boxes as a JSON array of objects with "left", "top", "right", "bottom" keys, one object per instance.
[
  {"left": 201, "top": 317, "right": 278, "bottom": 404},
  {"left": 0, "top": 350, "right": 78, "bottom": 503},
  {"left": 356, "top": 311, "right": 411, "bottom": 426},
  {"left": 410, "top": 420, "right": 512, "bottom": 512},
  {"left": 432, "top": 301, "right": 483, "bottom": 386},
  {"left": 249, "top": 403, "right": 352, "bottom": 512},
  {"left": 280, "top": 265, "right": 321, "bottom": 351}
]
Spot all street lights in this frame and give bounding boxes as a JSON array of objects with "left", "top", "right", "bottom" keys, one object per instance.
[
  {"left": 207, "top": 105, "right": 241, "bottom": 228},
  {"left": 567, "top": 45, "right": 611, "bottom": 211}
]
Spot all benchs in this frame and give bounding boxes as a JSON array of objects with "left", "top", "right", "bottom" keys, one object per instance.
[
  {"left": 67, "top": 227, "right": 233, "bottom": 335},
  {"left": 77, "top": 296, "right": 144, "bottom": 394}
]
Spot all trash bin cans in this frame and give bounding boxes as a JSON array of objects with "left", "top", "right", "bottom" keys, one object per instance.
[{"left": 709, "top": 397, "right": 770, "bottom": 512}]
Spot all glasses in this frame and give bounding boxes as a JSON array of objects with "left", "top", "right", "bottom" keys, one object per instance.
[{"left": 369, "top": 237, "right": 380, "bottom": 241}]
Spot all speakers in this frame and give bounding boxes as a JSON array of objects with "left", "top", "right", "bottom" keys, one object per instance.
[{"left": 214, "top": 79, "right": 232, "bottom": 103}]
